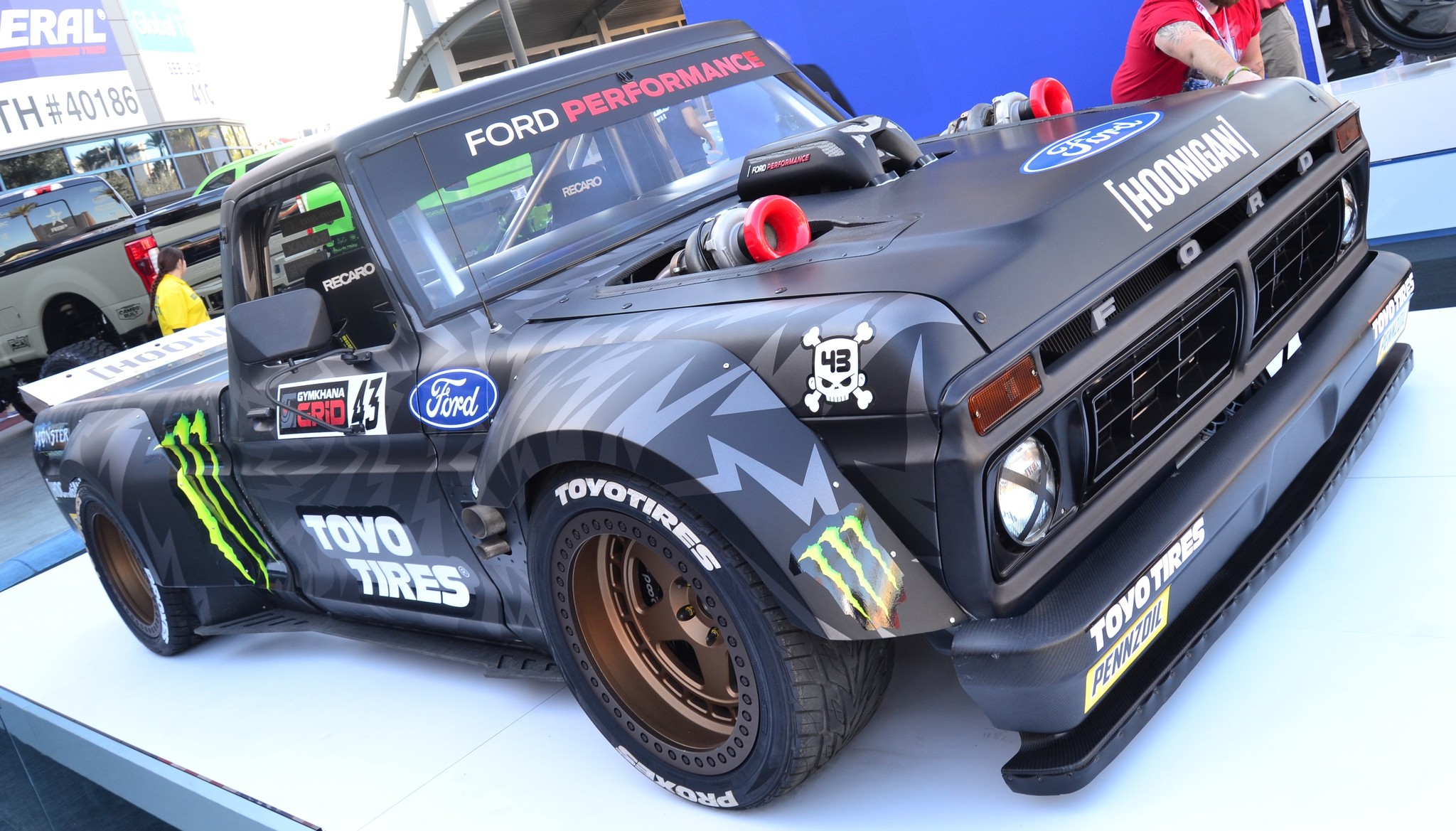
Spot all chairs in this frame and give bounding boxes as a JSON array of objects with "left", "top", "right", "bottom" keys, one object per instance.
[{"left": 308, "top": 249, "right": 400, "bottom": 354}]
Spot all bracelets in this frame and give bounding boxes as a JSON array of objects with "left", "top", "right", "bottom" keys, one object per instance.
[{"left": 1223, "top": 66, "right": 1251, "bottom": 86}]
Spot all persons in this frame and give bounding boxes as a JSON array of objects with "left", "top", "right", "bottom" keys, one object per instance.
[
  {"left": 651, "top": 97, "right": 716, "bottom": 176},
  {"left": 1343, "top": 0, "right": 1371, "bottom": 58},
  {"left": 146, "top": 245, "right": 211, "bottom": 337},
  {"left": 1333, "top": 0, "right": 1386, "bottom": 59},
  {"left": 1257, "top": 1, "right": 1308, "bottom": 80},
  {"left": 1110, "top": 0, "right": 1262, "bottom": 104}
]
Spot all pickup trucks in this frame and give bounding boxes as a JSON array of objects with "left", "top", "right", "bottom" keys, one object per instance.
[
  {"left": 11, "top": 19, "right": 1420, "bottom": 808},
  {"left": 1, "top": 173, "right": 232, "bottom": 424},
  {"left": 191, "top": 134, "right": 557, "bottom": 253}
]
